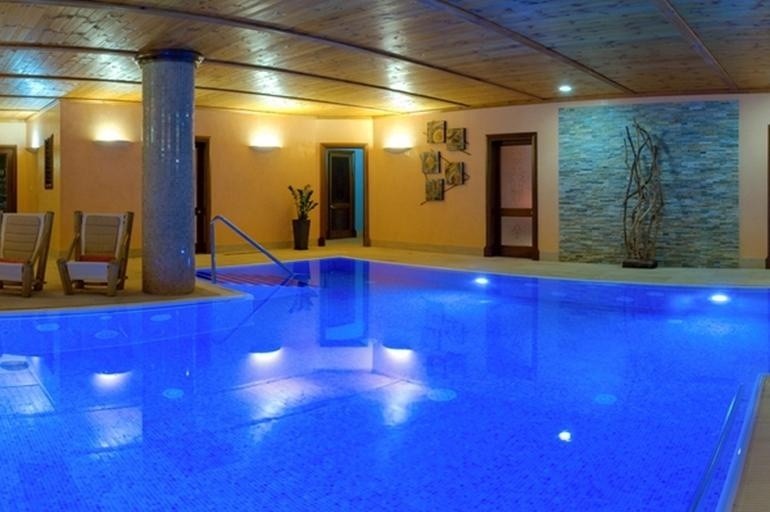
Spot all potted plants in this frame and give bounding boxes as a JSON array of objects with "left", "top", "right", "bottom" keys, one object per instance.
[
  {"left": 288, "top": 260, "right": 321, "bottom": 317},
  {"left": 286, "top": 182, "right": 320, "bottom": 252}
]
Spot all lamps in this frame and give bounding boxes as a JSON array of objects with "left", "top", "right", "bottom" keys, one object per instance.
[
  {"left": 248, "top": 142, "right": 283, "bottom": 155},
  {"left": 248, "top": 340, "right": 284, "bottom": 358},
  {"left": 93, "top": 354, "right": 133, "bottom": 378},
  {"left": 25, "top": 145, "right": 41, "bottom": 155},
  {"left": 85, "top": 135, "right": 141, "bottom": 155},
  {"left": 382, "top": 144, "right": 414, "bottom": 154},
  {"left": 381, "top": 334, "right": 413, "bottom": 355}
]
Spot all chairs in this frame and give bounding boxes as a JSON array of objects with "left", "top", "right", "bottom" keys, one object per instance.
[
  {"left": 0, "top": 208, "right": 56, "bottom": 294},
  {"left": 58, "top": 207, "right": 135, "bottom": 298}
]
